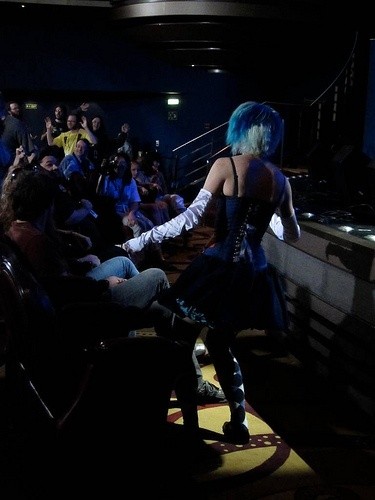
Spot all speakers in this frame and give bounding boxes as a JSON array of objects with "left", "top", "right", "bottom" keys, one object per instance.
[{"left": 334, "top": 143, "right": 370, "bottom": 167}]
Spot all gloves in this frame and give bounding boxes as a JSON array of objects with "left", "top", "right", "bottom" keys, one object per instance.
[
  {"left": 122, "top": 189, "right": 213, "bottom": 254},
  {"left": 269, "top": 213, "right": 302, "bottom": 243}
]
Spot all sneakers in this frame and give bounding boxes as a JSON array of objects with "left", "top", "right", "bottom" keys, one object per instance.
[{"left": 196, "top": 380, "right": 226, "bottom": 402}]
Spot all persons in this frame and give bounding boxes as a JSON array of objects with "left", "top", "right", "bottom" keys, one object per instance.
[
  {"left": 122, "top": 101, "right": 303, "bottom": 444},
  {"left": 0, "top": 100, "right": 39, "bottom": 178},
  {"left": 7, "top": 101, "right": 193, "bottom": 272},
  {"left": 0, "top": 173, "right": 227, "bottom": 403}
]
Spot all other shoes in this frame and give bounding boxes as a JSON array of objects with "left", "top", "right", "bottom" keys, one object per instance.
[{"left": 223, "top": 422, "right": 248, "bottom": 439}]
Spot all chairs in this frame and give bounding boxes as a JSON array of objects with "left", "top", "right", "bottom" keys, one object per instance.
[{"left": 0, "top": 243, "right": 103, "bottom": 424}]
